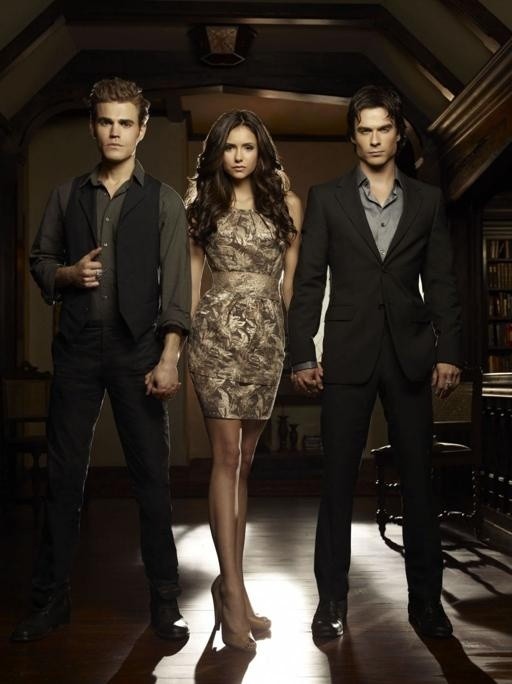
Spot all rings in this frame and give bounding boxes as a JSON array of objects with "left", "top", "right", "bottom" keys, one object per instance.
[
  {"left": 445, "top": 380, "right": 453, "bottom": 388},
  {"left": 95, "top": 269, "right": 101, "bottom": 279}
]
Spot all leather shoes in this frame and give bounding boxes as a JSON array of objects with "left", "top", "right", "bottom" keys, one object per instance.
[
  {"left": 405, "top": 590, "right": 454, "bottom": 640},
  {"left": 148, "top": 592, "right": 191, "bottom": 641},
  {"left": 12, "top": 578, "right": 78, "bottom": 643},
  {"left": 310, "top": 593, "right": 351, "bottom": 639}
]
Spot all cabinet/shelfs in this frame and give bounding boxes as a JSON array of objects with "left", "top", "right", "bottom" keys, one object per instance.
[{"left": 482, "top": 223, "right": 512, "bottom": 370}]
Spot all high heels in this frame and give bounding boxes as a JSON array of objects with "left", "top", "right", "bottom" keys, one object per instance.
[{"left": 208, "top": 572, "right": 272, "bottom": 654}]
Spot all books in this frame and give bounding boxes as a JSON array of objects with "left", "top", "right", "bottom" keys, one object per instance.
[{"left": 482, "top": 238, "right": 511, "bottom": 373}]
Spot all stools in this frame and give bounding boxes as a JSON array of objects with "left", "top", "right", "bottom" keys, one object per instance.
[{"left": 372, "top": 438, "right": 486, "bottom": 550}]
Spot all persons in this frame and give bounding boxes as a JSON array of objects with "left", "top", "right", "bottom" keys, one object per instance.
[
  {"left": 144, "top": 106, "right": 325, "bottom": 654},
  {"left": 283, "top": 81, "right": 461, "bottom": 644},
  {"left": 27, "top": 70, "right": 199, "bottom": 642}
]
